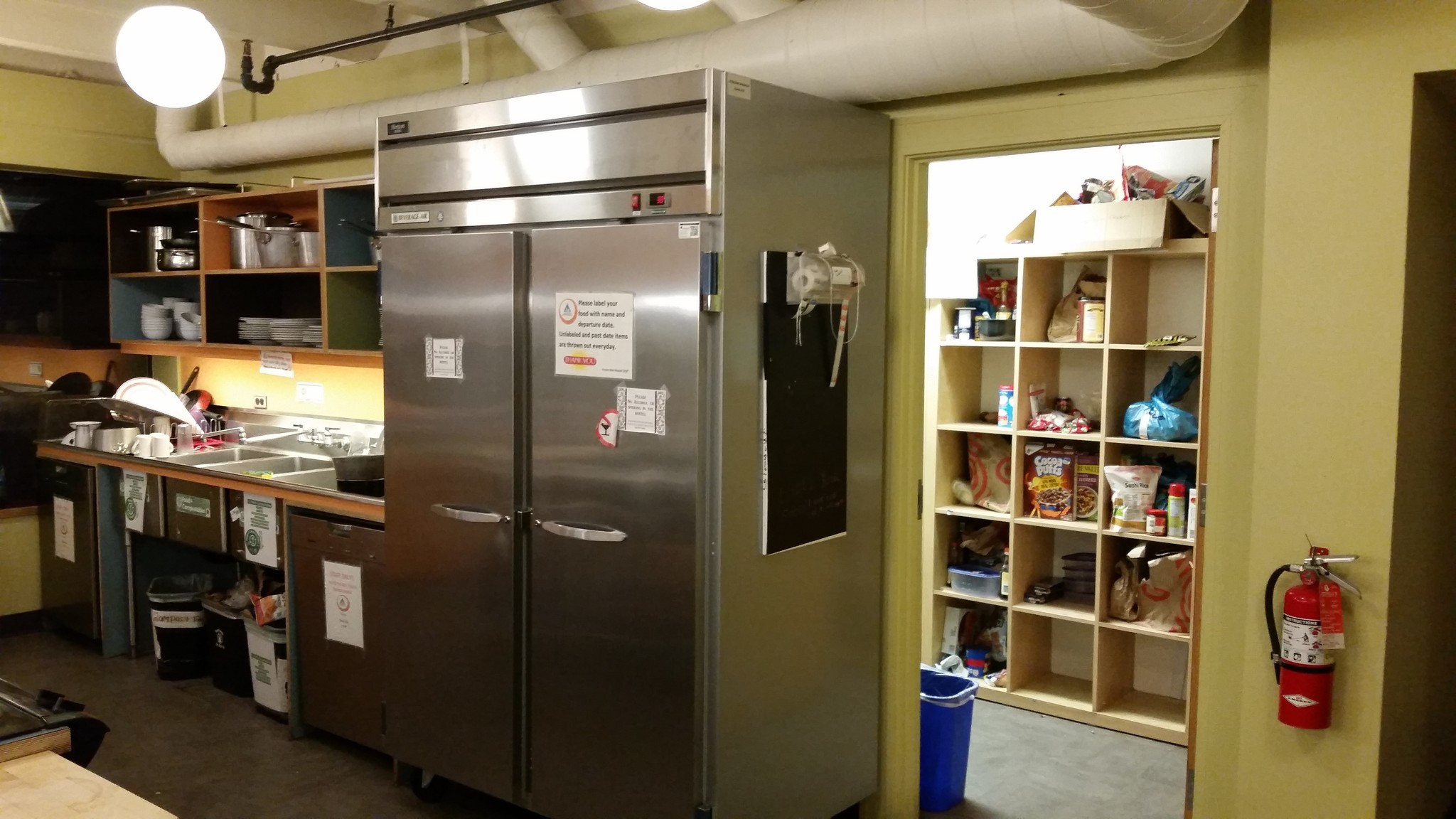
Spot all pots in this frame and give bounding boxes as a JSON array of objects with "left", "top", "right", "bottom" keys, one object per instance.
[
  {"left": 125, "top": 225, "right": 197, "bottom": 273},
  {"left": 93, "top": 421, "right": 140, "bottom": 455},
  {"left": 89, "top": 359, "right": 117, "bottom": 398},
  {"left": 193, "top": 211, "right": 321, "bottom": 268},
  {"left": 184, "top": 388, "right": 224, "bottom": 421}
]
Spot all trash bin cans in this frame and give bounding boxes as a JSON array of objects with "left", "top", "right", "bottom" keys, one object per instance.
[
  {"left": 145, "top": 572, "right": 213, "bottom": 681},
  {"left": 920, "top": 663, "right": 979, "bottom": 813},
  {"left": 239, "top": 607, "right": 290, "bottom": 726},
  {"left": 200, "top": 587, "right": 271, "bottom": 699}
]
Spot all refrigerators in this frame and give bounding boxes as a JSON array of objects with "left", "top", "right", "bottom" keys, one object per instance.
[{"left": 376, "top": 66, "right": 894, "bottom": 818}]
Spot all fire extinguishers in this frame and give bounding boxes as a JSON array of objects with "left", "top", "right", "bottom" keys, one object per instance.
[{"left": 1264, "top": 533, "right": 1364, "bottom": 730}]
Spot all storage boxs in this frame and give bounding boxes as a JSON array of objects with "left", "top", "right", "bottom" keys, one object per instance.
[{"left": 1006, "top": 190, "right": 1208, "bottom": 253}]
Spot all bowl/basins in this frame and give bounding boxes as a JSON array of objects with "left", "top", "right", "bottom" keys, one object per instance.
[{"left": 141, "top": 296, "right": 202, "bottom": 340}]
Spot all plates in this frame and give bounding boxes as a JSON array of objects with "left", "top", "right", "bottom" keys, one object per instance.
[{"left": 237, "top": 316, "right": 323, "bottom": 348}]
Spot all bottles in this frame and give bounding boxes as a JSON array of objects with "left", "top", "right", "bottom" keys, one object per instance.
[
  {"left": 999, "top": 547, "right": 1010, "bottom": 600},
  {"left": 1168, "top": 483, "right": 1185, "bottom": 539},
  {"left": 995, "top": 280, "right": 1012, "bottom": 319},
  {"left": 945, "top": 542, "right": 959, "bottom": 589}
]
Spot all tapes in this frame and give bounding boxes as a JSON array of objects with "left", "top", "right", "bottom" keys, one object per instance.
[
  {"left": 801, "top": 263, "right": 828, "bottom": 316},
  {"left": 829, "top": 264, "right": 864, "bottom": 387},
  {"left": 791, "top": 268, "right": 820, "bottom": 319}
]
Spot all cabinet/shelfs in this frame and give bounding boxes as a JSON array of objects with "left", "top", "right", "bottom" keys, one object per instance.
[
  {"left": 923, "top": 232, "right": 1209, "bottom": 755},
  {"left": 104, "top": 174, "right": 381, "bottom": 372}
]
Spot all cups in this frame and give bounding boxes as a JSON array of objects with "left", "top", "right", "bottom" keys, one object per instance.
[
  {"left": 131, "top": 432, "right": 174, "bottom": 458},
  {"left": 177, "top": 424, "right": 193, "bottom": 456},
  {"left": 75, "top": 425, "right": 92, "bottom": 450}
]
